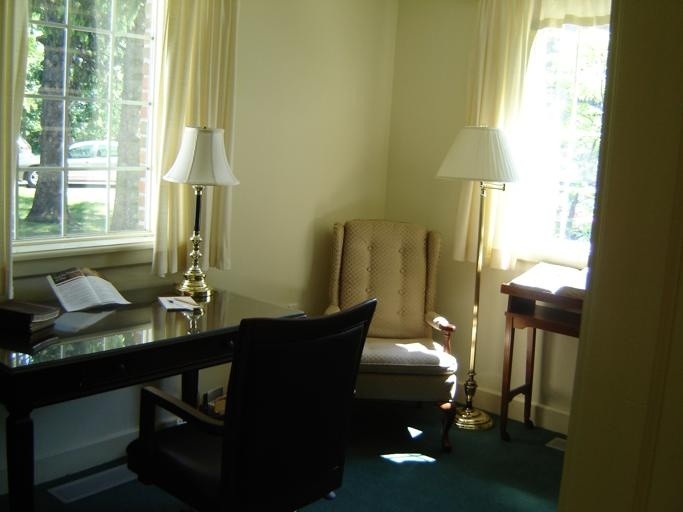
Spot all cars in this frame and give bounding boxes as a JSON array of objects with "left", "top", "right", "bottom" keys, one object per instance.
[{"left": 19, "top": 139, "right": 121, "bottom": 189}]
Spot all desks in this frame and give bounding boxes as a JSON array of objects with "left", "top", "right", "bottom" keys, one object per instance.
[
  {"left": 1, "top": 286, "right": 309, "bottom": 511},
  {"left": 500, "top": 263, "right": 585, "bottom": 440}
]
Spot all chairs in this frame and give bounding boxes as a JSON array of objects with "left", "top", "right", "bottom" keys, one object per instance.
[
  {"left": 322, "top": 220, "right": 458, "bottom": 454},
  {"left": 126, "top": 297, "right": 376, "bottom": 511}
]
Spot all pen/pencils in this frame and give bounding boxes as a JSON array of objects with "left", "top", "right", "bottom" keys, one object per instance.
[{"left": 176, "top": 299, "right": 194, "bottom": 305}]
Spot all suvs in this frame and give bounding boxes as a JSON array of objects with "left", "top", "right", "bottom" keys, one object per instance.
[{"left": 15, "top": 134, "right": 33, "bottom": 187}]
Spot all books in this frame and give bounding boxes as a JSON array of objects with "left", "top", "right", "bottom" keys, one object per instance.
[
  {"left": 0, "top": 300, "right": 60, "bottom": 323},
  {"left": 0, "top": 324, "right": 59, "bottom": 355},
  {"left": 47, "top": 263, "right": 133, "bottom": 313},
  {"left": 0, "top": 319, "right": 58, "bottom": 333},
  {"left": 511, "top": 257, "right": 590, "bottom": 301},
  {"left": 158, "top": 294, "right": 199, "bottom": 313}
]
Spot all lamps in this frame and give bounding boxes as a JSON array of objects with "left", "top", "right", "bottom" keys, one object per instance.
[
  {"left": 163, "top": 128, "right": 240, "bottom": 298},
  {"left": 436, "top": 125, "right": 516, "bottom": 429}
]
